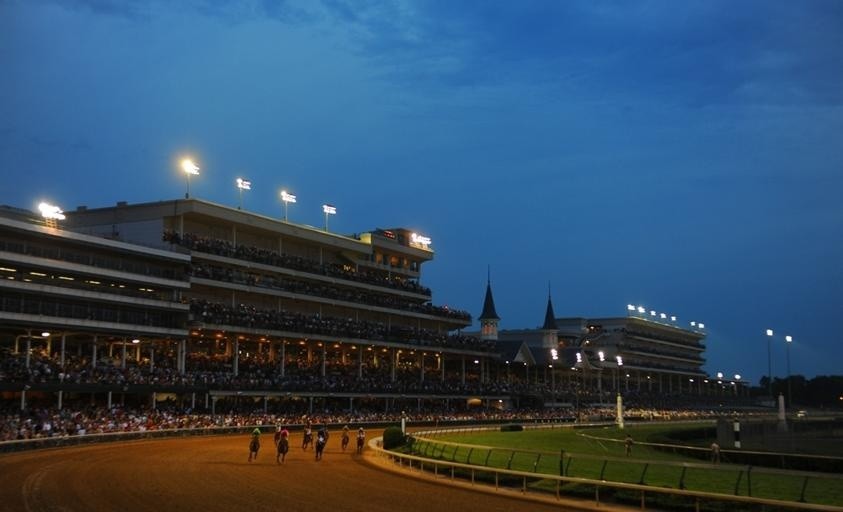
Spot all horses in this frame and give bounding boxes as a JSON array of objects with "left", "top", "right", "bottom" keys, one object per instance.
[
  {"left": 316, "top": 434, "right": 325, "bottom": 462},
  {"left": 357, "top": 437, "right": 364, "bottom": 454},
  {"left": 342, "top": 435, "right": 349, "bottom": 450},
  {"left": 302, "top": 430, "right": 313, "bottom": 450},
  {"left": 277, "top": 438, "right": 289, "bottom": 464},
  {"left": 249, "top": 438, "right": 260, "bottom": 463}
]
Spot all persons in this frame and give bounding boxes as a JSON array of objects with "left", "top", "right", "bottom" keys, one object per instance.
[
  {"left": 248, "top": 419, "right": 365, "bottom": 463},
  {"left": 508, "top": 326, "right": 777, "bottom": 422},
  {"left": 1, "top": 298, "right": 508, "bottom": 441},
  {"left": 710, "top": 440, "right": 721, "bottom": 463},
  {"left": 168, "top": 232, "right": 472, "bottom": 321},
  {"left": 624, "top": 434, "right": 636, "bottom": 457}
]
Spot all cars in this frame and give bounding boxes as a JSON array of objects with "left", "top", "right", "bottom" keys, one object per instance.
[{"left": 798, "top": 411, "right": 806, "bottom": 417}]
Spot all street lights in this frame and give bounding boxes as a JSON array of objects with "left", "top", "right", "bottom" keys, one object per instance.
[
  {"left": 181, "top": 156, "right": 199, "bottom": 198},
  {"left": 626, "top": 301, "right": 704, "bottom": 333},
  {"left": 765, "top": 330, "right": 774, "bottom": 398},
  {"left": 598, "top": 350, "right": 605, "bottom": 406},
  {"left": 615, "top": 355, "right": 623, "bottom": 395},
  {"left": 786, "top": 334, "right": 792, "bottom": 407},
  {"left": 281, "top": 190, "right": 337, "bottom": 232},
  {"left": 550, "top": 348, "right": 559, "bottom": 412},
  {"left": 233, "top": 176, "right": 250, "bottom": 205},
  {"left": 575, "top": 352, "right": 583, "bottom": 411}
]
